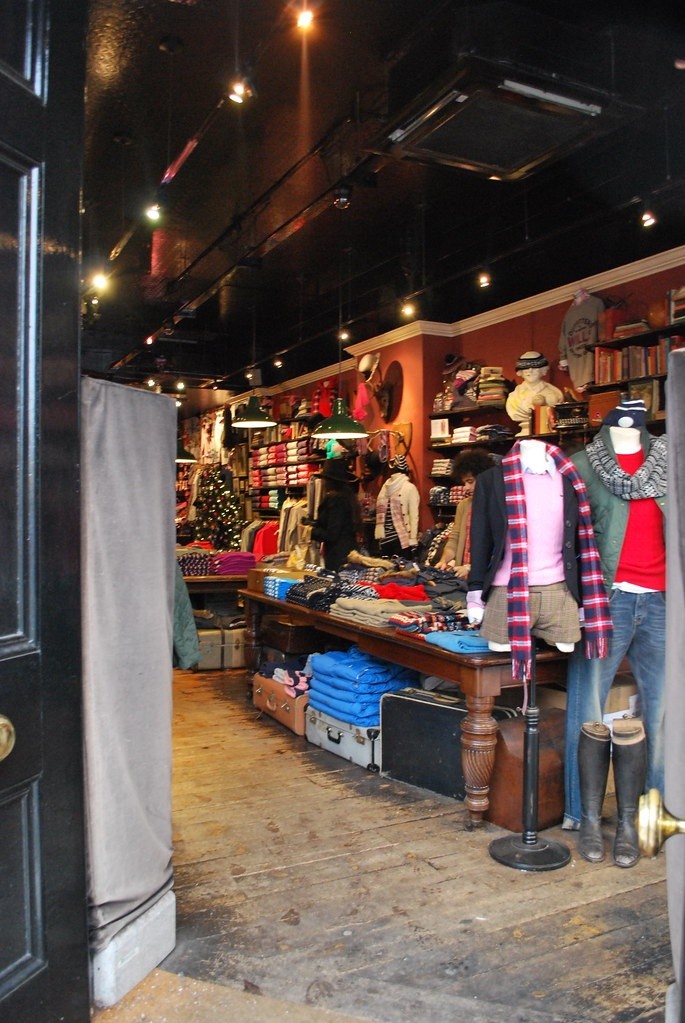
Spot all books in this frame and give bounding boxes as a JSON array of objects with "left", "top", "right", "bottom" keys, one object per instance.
[
  {"left": 595, "top": 336, "right": 685, "bottom": 385},
  {"left": 614, "top": 319, "right": 652, "bottom": 340},
  {"left": 629, "top": 379, "right": 659, "bottom": 422}
]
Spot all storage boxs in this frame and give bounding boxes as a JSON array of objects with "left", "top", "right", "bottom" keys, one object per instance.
[
  {"left": 249, "top": 568, "right": 317, "bottom": 592},
  {"left": 532, "top": 674, "right": 640, "bottom": 796},
  {"left": 589, "top": 389, "right": 628, "bottom": 428},
  {"left": 534, "top": 407, "right": 554, "bottom": 434}
]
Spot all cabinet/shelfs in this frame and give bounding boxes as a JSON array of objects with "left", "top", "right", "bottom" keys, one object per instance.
[{"left": 426, "top": 320, "right": 685, "bottom": 509}]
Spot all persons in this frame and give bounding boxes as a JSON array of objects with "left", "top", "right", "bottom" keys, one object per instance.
[
  {"left": 505, "top": 351, "right": 565, "bottom": 421},
  {"left": 465, "top": 438, "right": 614, "bottom": 680},
  {"left": 562, "top": 425, "right": 668, "bottom": 830},
  {"left": 374, "top": 472, "right": 420, "bottom": 562},
  {"left": 298, "top": 459, "right": 363, "bottom": 573},
  {"left": 434, "top": 448, "right": 495, "bottom": 580}
]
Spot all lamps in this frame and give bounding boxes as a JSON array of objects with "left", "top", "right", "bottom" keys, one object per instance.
[
  {"left": 310, "top": 190, "right": 367, "bottom": 440},
  {"left": 176, "top": 448, "right": 196, "bottom": 462},
  {"left": 231, "top": 282, "right": 278, "bottom": 429}
]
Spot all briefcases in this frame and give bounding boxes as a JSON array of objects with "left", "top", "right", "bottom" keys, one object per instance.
[
  {"left": 252, "top": 672, "right": 308, "bottom": 736},
  {"left": 303, "top": 704, "right": 382, "bottom": 773},
  {"left": 378, "top": 687, "right": 520, "bottom": 801}
]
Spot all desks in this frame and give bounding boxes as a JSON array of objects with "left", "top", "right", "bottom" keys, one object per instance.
[
  {"left": 237, "top": 586, "right": 634, "bottom": 831},
  {"left": 183, "top": 575, "right": 248, "bottom": 587}
]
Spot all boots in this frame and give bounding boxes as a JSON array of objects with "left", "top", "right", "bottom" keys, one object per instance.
[
  {"left": 577, "top": 726, "right": 613, "bottom": 862},
  {"left": 609, "top": 728, "right": 646, "bottom": 868}
]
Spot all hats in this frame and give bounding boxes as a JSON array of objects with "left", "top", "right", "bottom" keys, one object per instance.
[
  {"left": 312, "top": 460, "right": 358, "bottom": 485},
  {"left": 358, "top": 352, "right": 381, "bottom": 381},
  {"left": 603, "top": 399, "right": 646, "bottom": 426}
]
